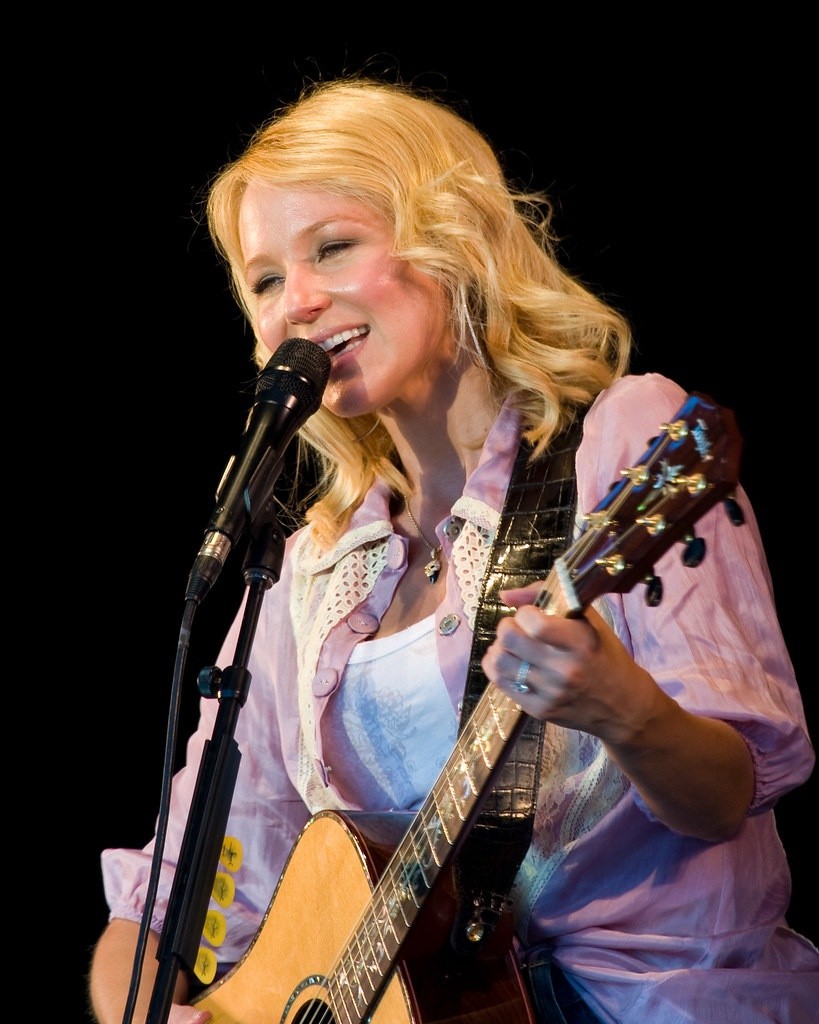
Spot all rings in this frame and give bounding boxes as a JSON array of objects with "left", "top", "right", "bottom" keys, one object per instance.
[{"left": 514, "top": 661, "right": 531, "bottom": 695}]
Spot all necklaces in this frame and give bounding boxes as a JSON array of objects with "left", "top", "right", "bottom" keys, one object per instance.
[{"left": 403, "top": 495, "right": 444, "bottom": 583}]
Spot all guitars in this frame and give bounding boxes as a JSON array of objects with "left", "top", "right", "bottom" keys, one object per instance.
[{"left": 185, "top": 392, "right": 744, "bottom": 1023}]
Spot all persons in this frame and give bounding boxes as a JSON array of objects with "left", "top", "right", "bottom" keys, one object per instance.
[{"left": 90, "top": 82, "right": 819, "bottom": 1024}]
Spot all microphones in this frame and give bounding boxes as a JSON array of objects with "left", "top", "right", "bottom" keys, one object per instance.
[{"left": 184, "top": 338, "right": 332, "bottom": 607}]
